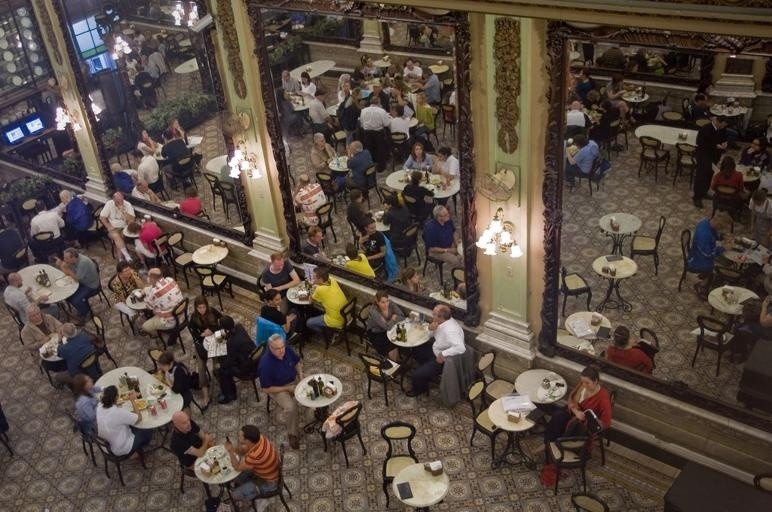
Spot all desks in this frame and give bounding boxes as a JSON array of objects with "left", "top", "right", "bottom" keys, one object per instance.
[{"left": 565, "top": 309, "right": 612, "bottom": 344}]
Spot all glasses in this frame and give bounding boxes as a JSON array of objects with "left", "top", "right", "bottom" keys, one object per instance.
[{"left": 274, "top": 345, "right": 286, "bottom": 351}]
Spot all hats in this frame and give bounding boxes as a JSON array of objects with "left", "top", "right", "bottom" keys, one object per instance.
[
  {"left": 62, "top": 323, "right": 78, "bottom": 338},
  {"left": 351, "top": 141, "right": 364, "bottom": 153}
]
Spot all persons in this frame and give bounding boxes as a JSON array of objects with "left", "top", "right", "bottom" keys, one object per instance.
[
  {"left": 259, "top": 334, "right": 304, "bottom": 450},
  {"left": 369, "top": 80, "right": 389, "bottom": 112},
  {"left": 391, "top": 74, "right": 410, "bottom": 107},
  {"left": 432, "top": 146, "right": 460, "bottom": 180},
  {"left": 599, "top": 42, "right": 665, "bottom": 75},
  {"left": 337, "top": 89, "right": 360, "bottom": 136},
  {"left": 261, "top": 251, "right": 300, "bottom": 315},
  {"left": 131, "top": 150, "right": 160, "bottom": 191},
  {"left": 135, "top": 129, "right": 156, "bottom": 159},
  {"left": 110, "top": 163, "right": 134, "bottom": 192},
  {"left": 261, "top": 288, "right": 296, "bottom": 344},
  {"left": 57, "top": 248, "right": 100, "bottom": 322},
  {"left": 21, "top": 303, "right": 63, "bottom": 366},
  {"left": 543, "top": 367, "right": 611, "bottom": 465},
  {"left": 357, "top": 219, "right": 386, "bottom": 278},
  {"left": 389, "top": 106, "right": 410, "bottom": 155},
  {"left": 742, "top": 136, "right": 771, "bottom": 171},
  {"left": 361, "top": 55, "right": 380, "bottom": 78},
  {"left": 281, "top": 70, "right": 301, "bottom": 94},
  {"left": 100, "top": 191, "right": 136, "bottom": 266},
  {"left": 170, "top": 412, "right": 214, "bottom": 470},
  {"left": 347, "top": 142, "right": 374, "bottom": 203},
  {"left": 307, "top": 90, "right": 334, "bottom": 144},
  {"left": 451, "top": 283, "right": 468, "bottom": 309},
  {"left": 142, "top": 268, "right": 185, "bottom": 345},
  {"left": 734, "top": 295, "right": 772, "bottom": 361},
  {"left": 422, "top": 206, "right": 461, "bottom": 270},
  {"left": 160, "top": 131, "right": 190, "bottom": 189},
  {"left": 132, "top": 180, "right": 161, "bottom": 205},
  {"left": 692, "top": 92, "right": 711, "bottom": 121},
  {"left": 155, "top": 351, "right": 193, "bottom": 412},
  {"left": 311, "top": 132, "right": 347, "bottom": 201},
  {"left": 28, "top": 200, "right": 64, "bottom": 264},
  {"left": 338, "top": 81, "right": 352, "bottom": 105},
  {"left": 59, "top": 190, "right": 90, "bottom": 249},
  {"left": 398, "top": 268, "right": 424, "bottom": 295},
  {"left": 565, "top": 101, "right": 592, "bottom": 135},
  {"left": 188, "top": 297, "right": 222, "bottom": 410},
  {"left": 607, "top": 326, "right": 654, "bottom": 376},
  {"left": 180, "top": 187, "right": 202, "bottom": 218},
  {"left": 404, "top": 59, "right": 423, "bottom": 81},
  {"left": 57, "top": 322, "right": 98, "bottom": 375},
  {"left": 0, "top": 214, "right": 28, "bottom": 282},
  {"left": 127, "top": 220, "right": 161, "bottom": 266},
  {"left": 112, "top": 261, "right": 148, "bottom": 338},
  {"left": 416, "top": 93, "right": 434, "bottom": 142},
  {"left": 405, "top": 16, "right": 444, "bottom": 48},
  {"left": 300, "top": 227, "right": 328, "bottom": 258},
  {"left": 406, "top": 304, "right": 465, "bottom": 397},
  {"left": 605, "top": 76, "right": 629, "bottom": 128},
  {"left": 350, "top": 72, "right": 366, "bottom": 90},
  {"left": 306, "top": 266, "right": 350, "bottom": 346},
  {"left": 382, "top": 195, "right": 411, "bottom": 239},
  {"left": 360, "top": 95, "right": 390, "bottom": 174},
  {"left": 412, "top": 68, "right": 441, "bottom": 104},
  {"left": 687, "top": 211, "right": 732, "bottom": 301},
  {"left": 163, "top": 116, "right": 183, "bottom": 143},
  {"left": 750, "top": 253, "right": 772, "bottom": 294},
  {"left": 96, "top": 386, "right": 151, "bottom": 463},
  {"left": 299, "top": 72, "right": 317, "bottom": 106},
  {"left": 225, "top": 424, "right": 281, "bottom": 511},
  {"left": 693, "top": 115, "right": 731, "bottom": 209},
  {"left": 711, "top": 156, "right": 744, "bottom": 220},
  {"left": 574, "top": 68, "right": 596, "bottom": 99},
  {"left": 3, "top": 273, "right": 49, "bottom": 324},
  {"left": 589, "top": 100, "right": 620, "bottom": 141},
  {"left": 565, "top": 135, "right": 599, "bottom": 183},
  {"left": 213, "top": 313, "right": 260, "bottom": 404},
  {"left": 343, "top": 244, "right": 376, "bottom": 279},
  {"left": 106, "top": 2, "right": 200, "bottom": 107},
  {"left": 367, "top": 290, "right": 405, "bottom": 357},
  {"left": 276, "top": 89, "right": 301, "bottom": 135},
  {"left": 344, "top": 189, "right": 373, "bottom": 226},
  {"left": 403, "top": 142, "right": 433, "bottom": 172},
  {"left": 70, "top": 374, "right": 100, "bottom": 435},
  {"left": 292, "top": 174, "right": 328, "bottom": 228}
]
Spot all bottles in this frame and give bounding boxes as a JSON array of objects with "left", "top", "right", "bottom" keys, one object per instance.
[
  {"left": 446, "top": 283, "right": 452, "bottom": 299},
  {"left": 135, "top": 384, "right": 142, "bottom": 399},
  {"left": 214, "top": 457, "right": 221, "bottom": 473},
  {"left": 225, "top": 436, "right": 233, "bottom": 444},
  {"left": 443, "top": 280, "right": 449, "bottom": 298},
  {"left": 318, "top": 377, "right": 324, "bottom": 396},
  {"left": 312, "top": 377, "right": 321, "bottom": 397},
  {"left": 396, "top": 324, "right": 401, "bottom": 341},
  {"left": 160, "top": 399, "right": 168, "bottom": 409},
  {"left": 125, "top": 373, "right": 132, "bottom": 389},
  {"left": 307, "top": 289, "right": 312, "bottom": 299},
  {"left": 150, "top": 405, "right": 157, "bottom": 418},
  {"left": 43, "top": 269, "right": 49, "bottom": 282},
  {"left": 401, "top": 324, "right": 407, "bottom": 343},
  {"left": 304, "top": 275, "right": 312, "bottom": 288},
  {"left": 426, "top": 169, "right": 430, "bottom": 184},
  {"left": 39, "top": 271, "right": 48, "bottom": 286},
  {"left": 641, "top": 82, "right": 647, "bottom": 100}
]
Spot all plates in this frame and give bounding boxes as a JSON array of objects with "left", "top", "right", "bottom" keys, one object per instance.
[
  {"left": 208, "top": 446, "right": 225, "bottom": 458},
  {"left": 548, "top": 389, "right": 562, "bottom": 397}
]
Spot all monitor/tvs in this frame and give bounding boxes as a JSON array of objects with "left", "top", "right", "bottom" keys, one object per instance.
[
  {"left": 4, "top": 126, "right": 25, "bottom": 143},
  {"left": 26, "top": 117, "right": 44, "bottom": 134}
]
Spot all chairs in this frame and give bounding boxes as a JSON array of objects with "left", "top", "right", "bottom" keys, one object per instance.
[
  {"left": 165, "top": 34, "right": 175, "bottom": 54},
  {"left": 638, "top": 136, "right": 670, "bottom": 181},
  {"left": 695, "top": 119, "right": 709, "bottom": 129},
  {"left": 326, "top": 122, "right": 346, "bottom": 152},
  {"left": 251, "top": 442, "right": 292, "bottom": 512},
  {"left": 545, "top": 435, "right": 590, "bottom": 496},
  {"left": 167, "top": 231, "right": 198, "bottom": 290},
  {"left": 21, "top": 198, "right": 39, "bottom": 219},
  {"left": 174, "top": 32, "right": 185, "bottom": 54},
  {"left": 155, "top": 175, "right": 170, "bottom": 202},
  {"left": 314, "top": 202, "right": 337, "bottom": 249},
  {"left": 639, "top": 327, "right": 661, "bottom": 359},
  {"left": 442, "top": 102, "right": 456, "bottom": 141},
  {"left": 140, "top": 79, "right": 158, "bottom": 106},
  {"left": 464, "top": 379, "right": 506, "bottom": 459},
  {"left": 203, "top": 172, "right": 228, "bottom": 215},
  {"left": 678, "top": 228, "right": 713, "bottom": 294},
  {"left": 362, "top": 163, "right": 382, "bottom": 210},
  {"left": 629, "top": 215, "right": 666, "bottom": 276},
  {"left": 319, "top": 402, "right": 367, "bottom": 469},
  {"left": 357, "top": 301, "right": 383, "bottom": 353},
  {"left": 32, "top": 231, "right": 58, "bottom": 254},
  {"left": 381, "top": 420, "right": 419, "bottom": 508},
  {"left": 475, "top": 348, "right": 515, "bottom": 400},
  {"left": 77, "top": 349, "right": 102, "bottom": 382},
  {"left": 421, "top": 228, "right": 449, "bottom": 287},
  {"left": 570, "top": 157, "right": 604, "bottom": 195},
  {"left": 662, "top": 111, "right": 683, "bottom": 127},
  {"left": 571, "top": 492, "right": 610, "bottom": 512},
  {"left": 316, "top": 172, "right": 348, "bottom": 215},
  {"left": 152, "top": 232, "right": 173, "bottom": 268},
  {"left": 404, "top": 223, "right": 421, "bottom": 269},
  {"left": 712, "top": 183, "right": 743, "bottom": 234},
  {"left": 161, "top": 430, "right": 193, "bottom": 495},
  {"left": 593, "top": 389, "right": 617, "bottom": 467},
  {"left": 84, "top": 256, "right": 111, "bottom": 315},
  {"left": 358, "top": 352, "right": 402, "bottom": 405},
  {"left": 391, "top": 132, "right": 405, "bottom": 171},
  {"left": 451, "top": 267, "right": 464, "bottom": 289},
  {"left": 171, "top": 155, "right": 198, "bottom": 193},
  {"left": 158, "top": 297, "right": 189, "bottom": 354},
  {"left": 323, "top": 296, "right": 357, "bottom": 357},
  {"left": 672, "top": 142, "right": 698, "bottom": 190},
  {"left": 65, "top": 408, "right": 100, "bottom": 466},
  {"left": 195, "top": 265, "right": 235, "bottom": 311},
  {"left": 85, "top": 203, "right": 107, "bottom": 250},
  {"left": 91, "top": 315, "right": 118, "bottom": 368},
  {"left": 11, "top": 244, "right": 30, "bottom": 270},
  {"left": 715, "top": 265, "right": 742, "bottom": 286},
  {"left": 691, "top": 313, "right": 736, "bottom": 377},
  {"left": 147, "top": 347, "right": 165, "bottom": 375},
  {"left": 559, "top": 265, "right": 591, "bottom": 317},
  {"left": 91, "top": 434, "right": 148, "bottom": 487},
  {"left": 217, "top": 180, "right": 242, "bottom": 221},
  {"left": 4, "top": 303, "right": 26, "bottom": 346},
  {"left": 247, "top": 340, "right": 274, "bottom": 413},
  {"left": 599, "top": 119, "right": 624, "bottom": 162}
]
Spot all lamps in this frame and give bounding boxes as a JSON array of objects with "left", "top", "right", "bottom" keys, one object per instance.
[
  {"left": 220, "top": 109, "right": 262, "bottom": 180},
  {"left": 471, "top": 163, "right": 523, "bottom": 263},
  {"left": 48, "top": 77, "right": 81, "bottom": 132},
  {"left": 171, "top": 1, "right": 201, "bottom": 29},
  {"left": 96, "top": 3, "right": 132, "bottom": 61}
]
